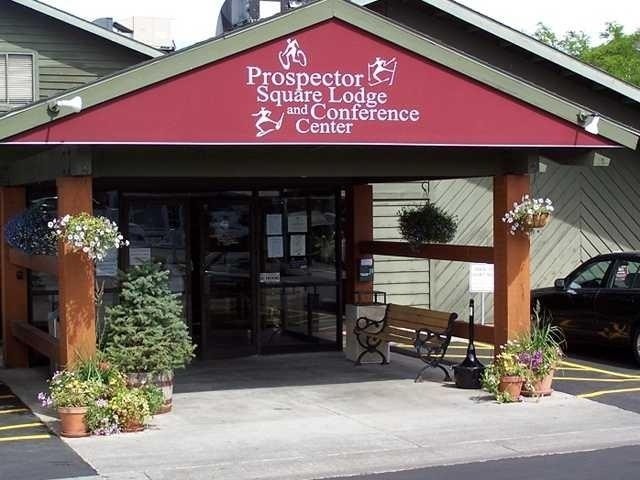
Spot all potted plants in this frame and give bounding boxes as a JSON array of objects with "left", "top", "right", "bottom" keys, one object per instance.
[
  {"left": 517, "top": 297, "right": 568, "bottom": 397},
  {"left": 110, "top": 384, "right": 164, "bottom": 433},
  {"left": 100, "top": 255, "right": 198, "bottom": 416}
]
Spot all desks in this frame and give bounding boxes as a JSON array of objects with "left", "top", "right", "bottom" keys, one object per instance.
[{"left": 205, "top": 269, "right": 346, "bottom": 356}]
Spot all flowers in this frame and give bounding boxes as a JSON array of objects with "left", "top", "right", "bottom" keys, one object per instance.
[
  {"left": 49, "top": 211, "right": 130, "bottom": 263},
  {"left": 478, "top": 339, "right": 543, "bottom": 404},
  {"left": 38, "top": 370, "right": 122, "bottom": 435},
  {"left": 501, "top": 193, "right": 555, "bottom": 237},
  {"left": 4, "top": 199, "right": 60, "bottom": 255}
]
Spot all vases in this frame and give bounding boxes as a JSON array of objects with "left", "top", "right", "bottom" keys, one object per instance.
[
  {"left": 522, "top": 211, "right": 549, "bottom": 228},
  {"left": 56, "top": 405, "right": 92, "bottom": 438},
  {"left": 58, "top": 239, "right": 79, "bottom": 257},
  {"left": 499, "top": 375, "right": 524, "bottom": 402}
]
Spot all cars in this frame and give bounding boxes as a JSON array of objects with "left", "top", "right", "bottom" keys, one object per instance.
[
  {"left": 531, "top": 251, "right": 640, "bottom": 364},
  {"left": 30, "top": 195, "right": 121, "bottom": 219}
]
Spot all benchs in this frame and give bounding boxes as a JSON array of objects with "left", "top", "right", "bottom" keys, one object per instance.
[{"left": 353, "top": 303, "right": 457, "bottom": 384}]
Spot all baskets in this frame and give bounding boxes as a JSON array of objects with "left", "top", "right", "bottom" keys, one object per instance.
[{"left": 512, "top": 212, "right": 549, "bottom": 229}]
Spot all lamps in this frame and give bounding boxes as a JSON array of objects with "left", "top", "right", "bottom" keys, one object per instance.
[
  {"left": 47, "top": 96, "right": 82, "bottom": 114},
  {"left": 577, "top": 110, "right": 601, "bottom": 135}
]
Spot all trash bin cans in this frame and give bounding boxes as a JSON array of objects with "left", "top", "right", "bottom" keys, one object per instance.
[
  {"left": 345, "top": 291, "right": 391, "bottom": 366},
  {"left": 47, "top": 311, "right": 59, "bottom": 373}
]
